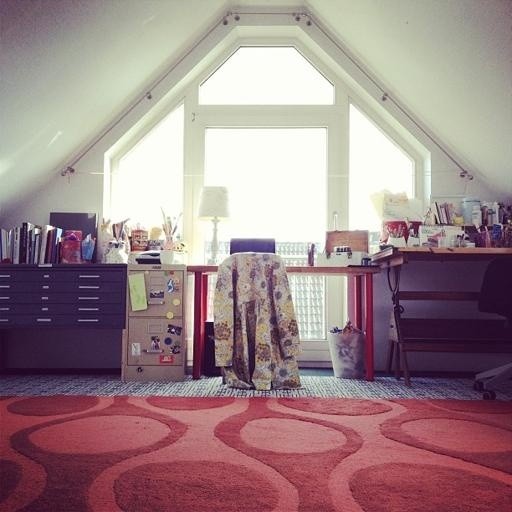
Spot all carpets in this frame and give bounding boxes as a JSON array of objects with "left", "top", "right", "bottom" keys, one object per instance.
[{"left": 1, "top": 394, "right": 511, "bottom": 511}]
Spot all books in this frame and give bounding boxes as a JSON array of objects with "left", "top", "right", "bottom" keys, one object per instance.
[
  {"left": 431, "top": 201, "right": 454, "bottom": 224},
  {"left": 0, "top": 222, "right": 62, "bottom": 264}
]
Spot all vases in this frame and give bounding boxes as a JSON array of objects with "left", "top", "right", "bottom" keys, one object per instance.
[{"left": 160, "top": 250, "right": 174, "bottom": 264}]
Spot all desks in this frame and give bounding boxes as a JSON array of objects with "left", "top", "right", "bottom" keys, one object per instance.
[
  {"left": 187, "top": 264, "right": 381, "bottom": 384},
  {"left": 370, "top": 247, "right": 512, "bottom": 390}
]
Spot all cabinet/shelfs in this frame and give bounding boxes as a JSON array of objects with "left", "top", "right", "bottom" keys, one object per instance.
[
  {"left": 0, "top": 264, "right": 127, "bottom": 330},
  {"left": 125, "top": 266, "right": 185, "bottom": 382}
]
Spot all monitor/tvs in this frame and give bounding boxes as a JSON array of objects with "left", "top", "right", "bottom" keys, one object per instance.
[{"left": 231, "top": 238, "right": 275, "bottom": 253}]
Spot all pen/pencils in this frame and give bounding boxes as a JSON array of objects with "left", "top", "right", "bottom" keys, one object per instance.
[{"left": 160, "top": 207, "right": 183, "bottom": 241}]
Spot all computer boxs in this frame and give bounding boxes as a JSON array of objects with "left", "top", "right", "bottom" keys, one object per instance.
[{"left": 204, "top": 322, "right": 222, "bottom": 377}]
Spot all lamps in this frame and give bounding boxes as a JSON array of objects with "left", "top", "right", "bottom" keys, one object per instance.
[{"left": 194, "top": 185, "right": 231, "bottom": 264}]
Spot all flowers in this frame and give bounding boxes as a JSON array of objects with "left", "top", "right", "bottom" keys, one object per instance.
[{"left": 157, "top": 207, "right": 183, "bottom": 249}]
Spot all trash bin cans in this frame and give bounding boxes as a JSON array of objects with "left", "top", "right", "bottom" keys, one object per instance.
[{"left": 326, "top": 331, "right": 366, "bottom": 379}]
